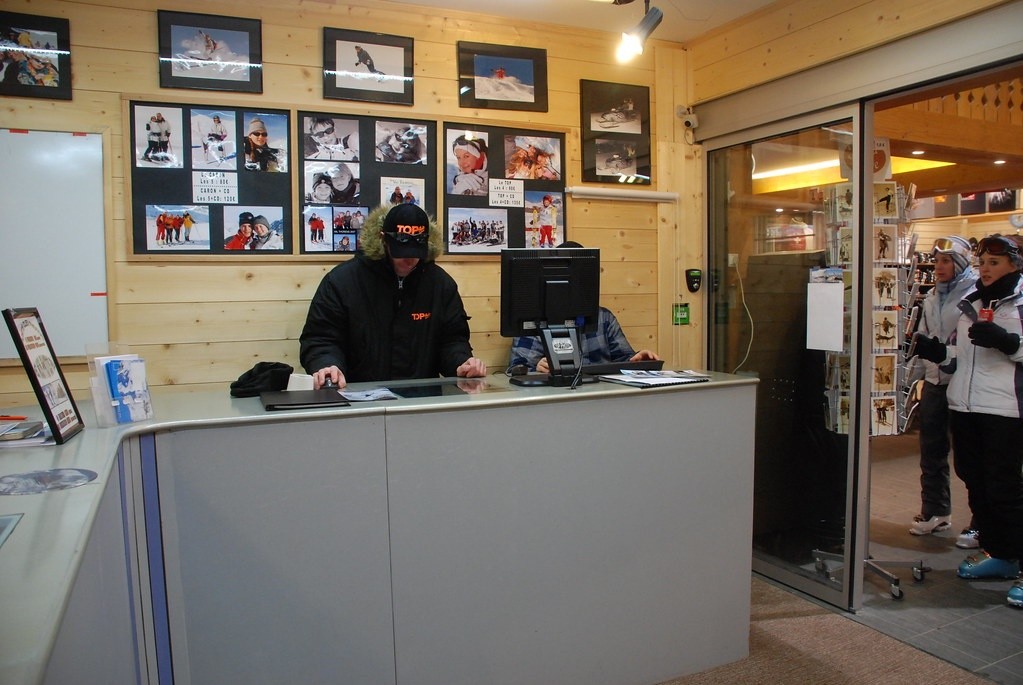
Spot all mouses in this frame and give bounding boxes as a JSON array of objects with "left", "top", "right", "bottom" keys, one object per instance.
[
  {"left": 510, "top": 364, "right": 528, "bottom": 376},
  {"left": 320, "top": 375, "right": 339, "bottom": 390}
]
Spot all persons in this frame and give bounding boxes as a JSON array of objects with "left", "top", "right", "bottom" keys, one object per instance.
[
  {"left": 374, "top": 127, "right": 428, "bottom": 165},
  {"left": 505, "top": 241, "right": 661, "bottom": 377},
  {"left": 298, "top": 203, "right": 488, "bottom": 392},
  {"left": 303, "top": 116, "right": 359, "bottom": 161},
  {"left": 308, "top": 210, "right": 365, "bottom": 251},
  {"left": 840, "top": 187, "right": 894, "bottom": 425},
  {"left": 354, "top": 45, "right": 385, "bottom": 83},
  {"left": 908, "top": 235, "right": 980, "bottom": 548},
  {"left": 490, "top": 67, "right": 505, "bottom": 78},
  {"left": 0, "top": 25, "right": 61, "bottom": 86},
  {"left": 903, "top": 233, "right": 1023, "bottom": 606},
  {"left": 143, "top": 113, "right": 284, "bottom": 249},
  {"left": 452, "top": 133, "right": 488, "bottom": 196},
  {"left": 198, "top": 30, "right": 216, "bottom": 59},
  {"left": 532, "top": 194, "right": 555, "bottom": 248},
  {"left": 449, "top": 216, "right": 506, "bottom": 247},
  {"left": 389, "top": 187, "right": 416, "bottom": 205},
  {"left": 305, "top": 162, "right": 362, "bottom": 206}
]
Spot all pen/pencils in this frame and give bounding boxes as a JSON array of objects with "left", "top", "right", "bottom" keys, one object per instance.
[{"left": 0, "top": 415, "right": 28, "bottom": 421}]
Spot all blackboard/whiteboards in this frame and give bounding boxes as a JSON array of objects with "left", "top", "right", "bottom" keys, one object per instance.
[{"left": 1, "top": 122, "right": 118, "bottom": 366}]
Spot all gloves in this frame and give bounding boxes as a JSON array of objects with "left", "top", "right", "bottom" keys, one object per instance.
[
  {"left": 152, "top": 132, "right": 170, "bottom": 138},
  {"left": 914, "top": 333, "right": 946, "bottom": 364},
  {"left": 356, "top": 63, "right": 360, "bottom": 66},
  {"left": 968, "top": 321, "right": 1020, "bottom": 356}
]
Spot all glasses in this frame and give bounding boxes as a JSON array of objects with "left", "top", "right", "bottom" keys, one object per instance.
[
  {"left": 452, "top": 139, "right": 480, "bottom": 153},
  {"left": 32, "top": 57, "right": 48, "bottom": 65},
  {"left": 974, "top": 237, "right": 1010, "bottom": 257},
  {"left": 240, "top": 215, "right": 263, "bottom": 221},
  {"left": 251, "top": 131, "right": 268, "bottom": 137},
  {"left": 395, "top": 132, "right": 411, "bottom": 149},
  {"left": 930, "top": 237, "right": 956, "bottom": 256},
  {"left": 313, "top": 127, "right": 334, "bottom": 138},
  {"left": 385, "top": 231, "right": 430, "bottom": 244}
]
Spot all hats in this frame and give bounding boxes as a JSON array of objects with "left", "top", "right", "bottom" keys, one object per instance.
[
  {"left": 248, "top": 117, "right": 266, "bottom": 136},
  {"left": 355, "top": 45, "right": 361, "bottom": 49},
  {"left": 239, "top": 212, "right": 270, "bottom": 231},
  {"left": 312, "top": 163, "right": 352, "bottom": 191},
  {"left": 944, "top": 235, "right": 972, "bottom": 270},
  {"left": 383, "top": 203, "right": 429, "bottom": 258}
]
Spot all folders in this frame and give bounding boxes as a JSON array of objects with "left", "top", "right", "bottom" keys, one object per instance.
[{"left": 259, "top": 388, "right": 352, "bottom": 412}]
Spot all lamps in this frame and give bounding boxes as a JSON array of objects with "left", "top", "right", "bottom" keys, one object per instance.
[{"left": 615, "top": 0, "right": 664, "bottom": 64}]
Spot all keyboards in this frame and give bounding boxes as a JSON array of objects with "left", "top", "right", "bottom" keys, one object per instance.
[{"left": 560, "top": 359, "right": 664, "bottom": 376}]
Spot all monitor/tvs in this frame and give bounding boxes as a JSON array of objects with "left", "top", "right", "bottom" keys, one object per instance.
[
  {"left": 501, "top": 248, "right": 600, "bottom": 386},
  {"left": 381, "top": 378, "right": 518, "bottom": 397}
]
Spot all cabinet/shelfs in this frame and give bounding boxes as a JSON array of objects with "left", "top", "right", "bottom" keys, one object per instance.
[{"left": 812, "top": 136, "right": 932, "bottom": 600}]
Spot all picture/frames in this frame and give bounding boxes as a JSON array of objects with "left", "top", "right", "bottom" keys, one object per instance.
[
  {"left": 324, "top": 27, "right": 414, "bottom": 106},
  {"left": 121, "top": 91, "right": 567, "bottom": 264},
  {"left": 158, "top": 10, "right": 264, "bottom": 94},
  {"left": 581, "top": 78, "right": 652, "bottom": 186},
  {"left": 457, "top": 40, "right": 548, "bottom": 113},
  {"left": 0, "top": 10, "right": 72, "bottom": 100},
  {"left": 1, "top": 307, "right": 85, "bottom": 445}
]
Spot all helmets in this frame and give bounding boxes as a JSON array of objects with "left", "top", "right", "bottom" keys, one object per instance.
[
  {"left": 543, "top": 195, "right": 552, "bottom": 204},
  {"left": 213, "top": 115, "right": 220, "bottom": 119}
]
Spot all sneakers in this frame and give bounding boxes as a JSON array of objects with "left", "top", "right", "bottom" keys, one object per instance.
[
  {"left": 1006, "top": 580, "right": 1023, "bottom": 605},
  {"left": 909, "top": 512, "right": 955, "bottom": 535},
  {"left": 955, "top": 528, "right": 980, "bottom": 548},
  {"left": 954, "top": 547, "right": 1022, "bottom": 580}
]
